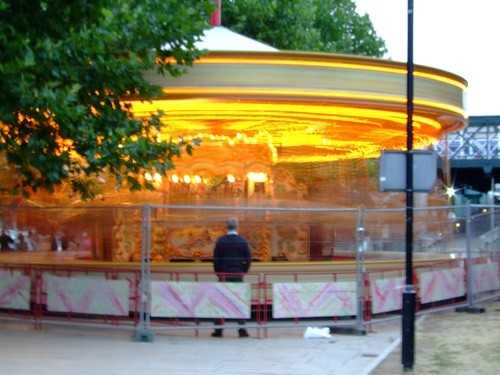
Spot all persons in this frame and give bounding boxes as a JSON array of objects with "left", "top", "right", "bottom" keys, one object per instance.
[
  {"left": 210, "top": 218, "right": 252, "bottom": 337},
  {"left": 1, "top": 226, "right": 89, "bottom": 253}
]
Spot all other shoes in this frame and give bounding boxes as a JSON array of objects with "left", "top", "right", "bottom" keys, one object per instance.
[
  {"left": 210, "top": 331, "right": 223, "bottom": 337},
  {"left": 238, "top": 328, "right": 249, "bottom": 337}
]
